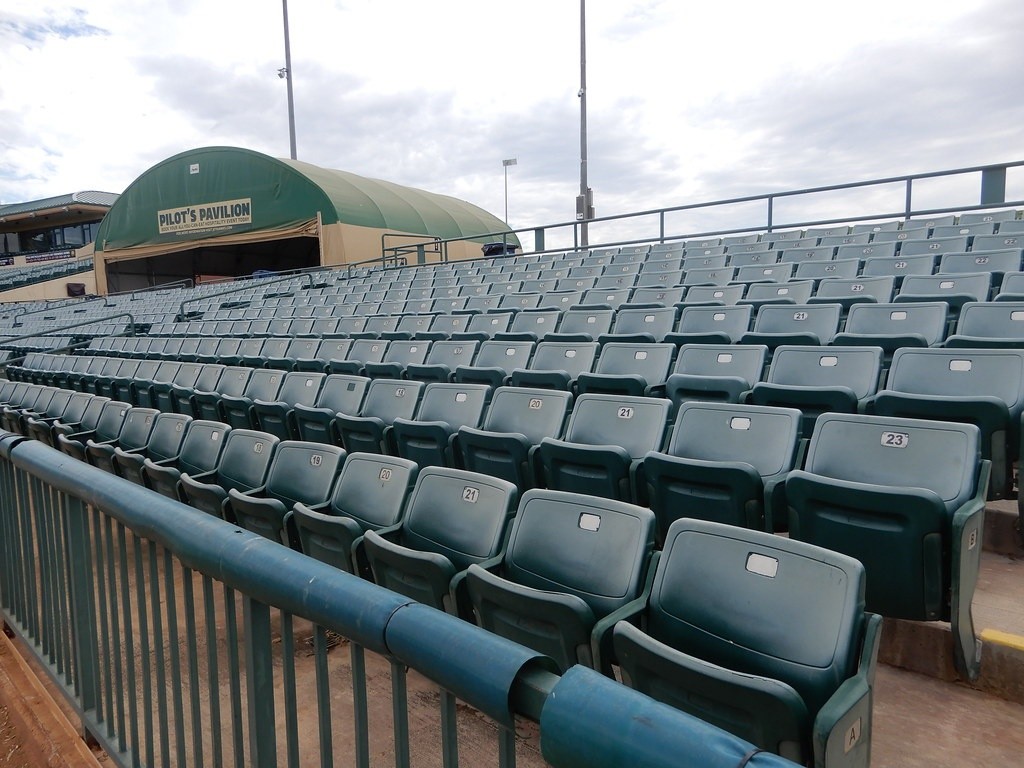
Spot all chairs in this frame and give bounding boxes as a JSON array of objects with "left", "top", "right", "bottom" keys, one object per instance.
[{"left": 0, "top": 209, "right": 1024, "bottom": 768}]
